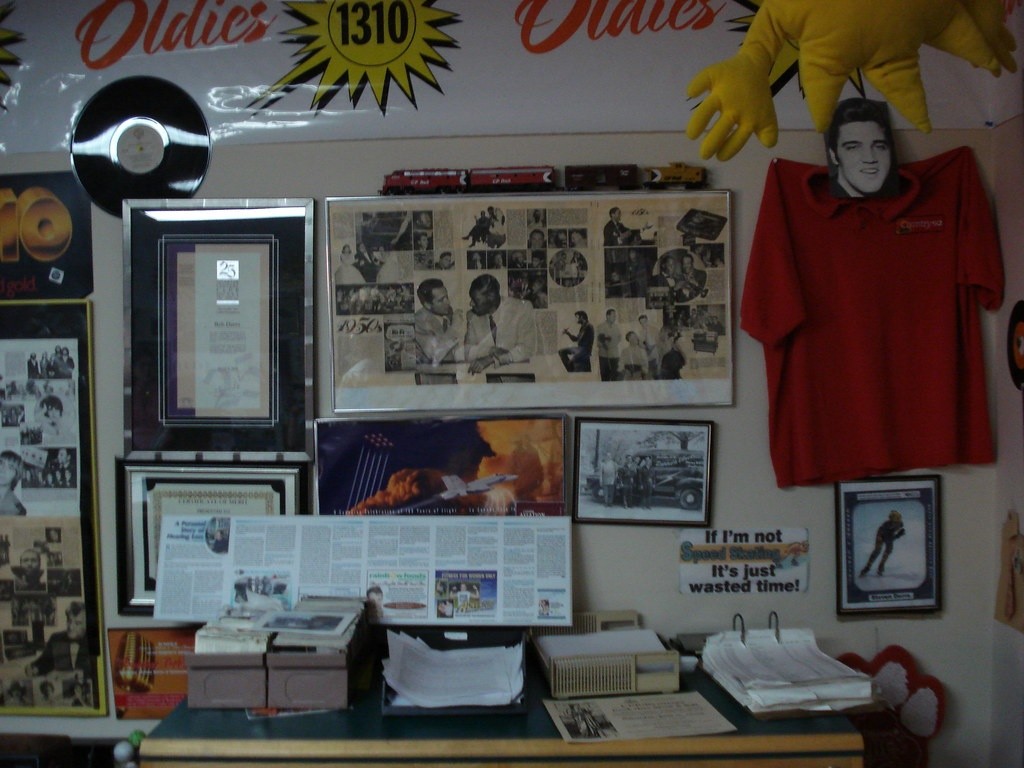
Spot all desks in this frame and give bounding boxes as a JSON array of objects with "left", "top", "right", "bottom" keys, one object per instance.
[{"left": 137, "top": 658, "right": 863, "bottom": 768}]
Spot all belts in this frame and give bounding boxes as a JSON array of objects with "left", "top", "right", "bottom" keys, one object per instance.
[{"left": 443, "top": 318, "right": 447, "bottom": 332}]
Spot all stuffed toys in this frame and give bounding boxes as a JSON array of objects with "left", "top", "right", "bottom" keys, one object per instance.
[{"left": 685, "top": 0, "right": 1018, "bottom": 162}]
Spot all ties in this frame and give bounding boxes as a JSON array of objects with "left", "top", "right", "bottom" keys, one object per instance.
[{"left": 489, "top": 315, "right": 497, "bottom": 345}]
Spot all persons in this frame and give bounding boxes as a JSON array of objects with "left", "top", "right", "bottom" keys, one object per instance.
[
  {"left": 857, "top": 508, "right": 907, "bottom": 580},
  {"left": 826, "top": 97, "right": 903, "bottom": 201},
  {"left": 1, "top": 196, "right": 727, "bottom": 716}
]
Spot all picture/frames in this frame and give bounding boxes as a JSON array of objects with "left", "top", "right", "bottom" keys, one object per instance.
[
  {"left": 0, "top": 299, "right": 108, "bottom": 716},
  {"left": 836, "top": 476, "right": 945, "bottom": 617},
  {"left": 120, "top": 197, "right": 317, "bottom": 466},
  {"left": 325, "top": 191, "right": 736, "bottom": 409},
  {"left": 116, "top": 457, "right": 309, "bottom": 617},
  {"left": 573, "top": 413, "right": 715, "bottom": 529}
]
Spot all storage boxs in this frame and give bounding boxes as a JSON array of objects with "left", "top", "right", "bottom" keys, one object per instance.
[
  {"left": 185, "top": 651, "right": 266, "bottom": 711},
  {"left": 267, "top": 632, "right": 381, "bottom": 712},
  {"left": 530, "top": 609, "right": 681, "bottom": 699}
]
